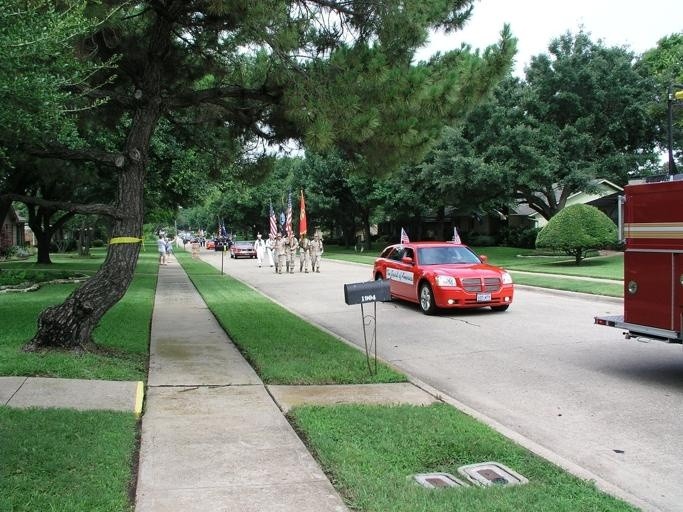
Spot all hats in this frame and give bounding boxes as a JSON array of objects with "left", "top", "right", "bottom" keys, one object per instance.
[{"left": 257, "top": 234, "right": 262, "bottom": 237}]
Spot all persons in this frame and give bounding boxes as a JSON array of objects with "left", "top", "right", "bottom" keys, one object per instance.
[
  {"left": 216, "top": 232, "right": 237, "bottom": 252},
  {"left": 426, "top": 228, "right": 435, "bottom": 240},
  {"left": 265, "top": 233, "right": 275, "bottom": 268},
  {"left": 310, "top": 232, "right": 323, "bottom": 273},
  {"left": 285, "top": 234, "right": 298, "bottom": 274},
  {"left": 165, "top": 238, "right": 176, "bottom": 263},
  {"left": 253, "top": 234, "right": 265, "bottom": 268},
  {"left": 275, "top": 233, "right": 289, "bottom": 274},
  {"left": 298, "top": 234, "right": 311, "bottom": 273},
  {"left": 157, "top": 235, "right": 166, "bottom": 266},
  {"left": 181, "top": 234, "right": 207, "bottom": 260}
]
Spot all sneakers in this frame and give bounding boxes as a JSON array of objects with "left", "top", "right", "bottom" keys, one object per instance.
[{"left": 257, "top": 265, "right": 321, "bottom": 273}]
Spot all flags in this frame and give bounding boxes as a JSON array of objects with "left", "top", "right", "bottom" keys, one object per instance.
[
  {"left": 278, "top": 198, "right": 286, "bottom": 237},
  {"left": 268, "top": 198, "right": 277, "bottom": 239},
  {"left": 298, "top": 193, "right": 308, "bottom": 236},
  {"left": 401, "top": 228, "right": 409, "bottom": 244},
  {"left": 197, "top": 219, "right": 226, "bottom": 239},
  {"left": 285, "top": 187, "right": 293, "bottom": 236},
  {"left": 452, "top": 227, "right": 461, "bottom": 242}
]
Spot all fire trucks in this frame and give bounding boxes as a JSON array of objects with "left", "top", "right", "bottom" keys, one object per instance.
[{"left": 594, "top": 83, "right": 683, "bottom": 344}]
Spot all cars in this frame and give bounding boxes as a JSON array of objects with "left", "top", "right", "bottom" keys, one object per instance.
[
  {"left": 373, "top": 241, "right": 513, "bottom": 315},
  {"left": 181, "top": 232, "right": 201, "bottom": 244},
  {"left": 206, "top": 237, "right": 257, "bottom": 259}
]
